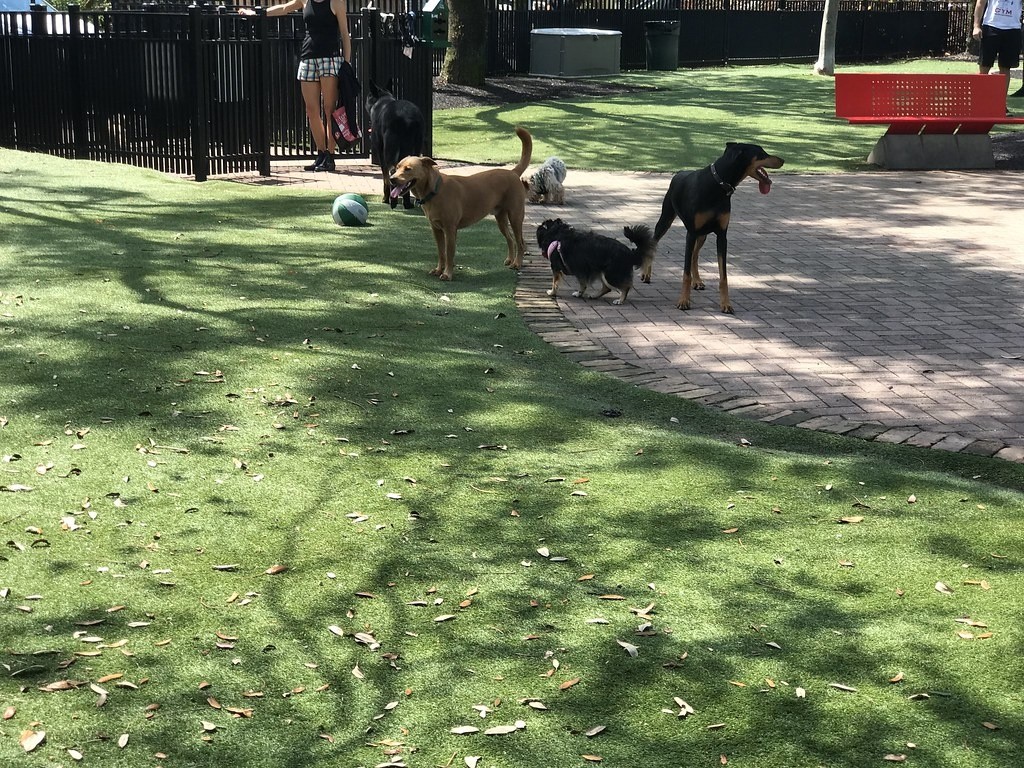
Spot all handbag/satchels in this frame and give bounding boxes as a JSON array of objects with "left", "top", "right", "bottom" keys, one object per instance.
[{"left": 331, "top": 105, "right": 363, "bottom": 150}]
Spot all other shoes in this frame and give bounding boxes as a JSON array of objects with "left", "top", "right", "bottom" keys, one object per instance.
[{"left": 1006, "top": 106, "right": 1012, "bottom": 117}]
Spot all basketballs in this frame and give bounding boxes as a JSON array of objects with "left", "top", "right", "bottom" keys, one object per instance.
[{"left": 331, "top": 193, "right": 366, "bottom": 226}]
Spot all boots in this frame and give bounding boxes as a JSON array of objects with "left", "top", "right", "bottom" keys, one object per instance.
[
  {"left": 303, "top": 150, "right": 326, "bottom": 171},
  {"left": 314, "top": 150, "right": 336, "bottom": 171}
]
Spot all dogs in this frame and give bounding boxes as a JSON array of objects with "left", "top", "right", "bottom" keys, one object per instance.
[
  {"left": 536, "top": 218, "right": 658, "bottom": 305},
  {"left": 363, "top": 75, "right": 425, "bottom": 211},
  {"left": 640, "top": 142, "right": 785, "bottom": 314},
  {"left": 387, "top": 125, "right": 533, "bottom": 282},
  {"left": 520, "top": 155, "right": 567, "bottom": 206}
]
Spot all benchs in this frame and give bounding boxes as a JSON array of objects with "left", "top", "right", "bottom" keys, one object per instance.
[{"left": 833, "top": 74, "right": 1024, "bottom": 135}]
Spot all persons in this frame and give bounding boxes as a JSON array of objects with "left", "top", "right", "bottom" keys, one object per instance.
[
  {"left": 237, "top": 0, "right": 351, "bottom": 172},
  {"left": 973, "top": 0, "right": 1024, "bottom": 117}
]
